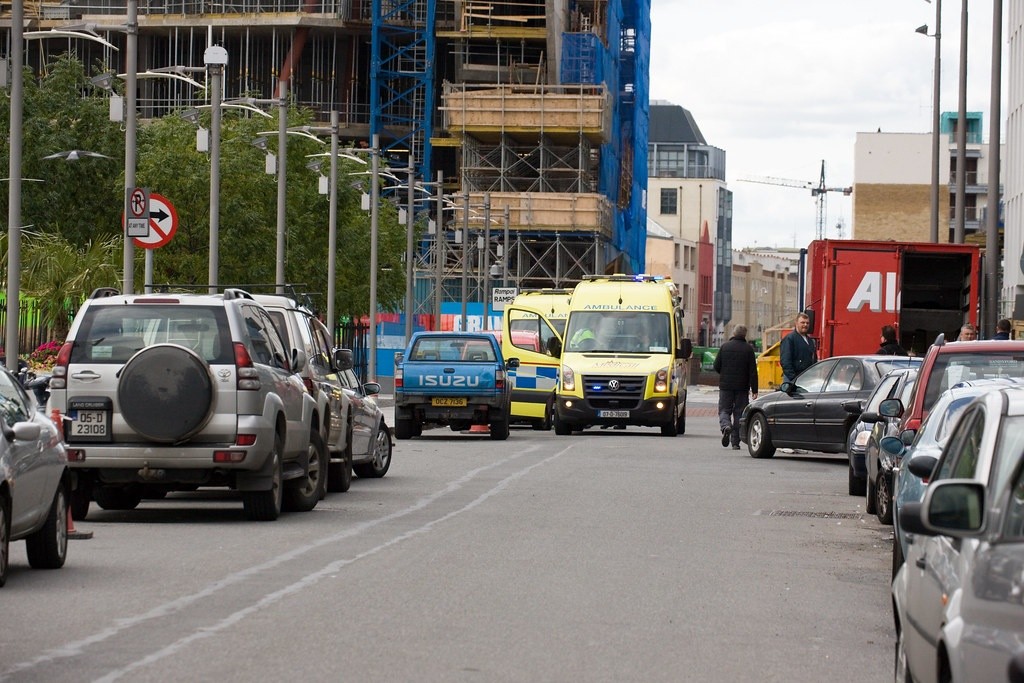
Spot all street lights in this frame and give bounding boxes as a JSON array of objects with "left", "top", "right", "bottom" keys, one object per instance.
[
  {"left": 301, "top": 130, "right": 380, "bottom": 392},
  {"left": 183, "top": 76, "right": 287, "bottom": 294},
  {"left": 4, "top": 31, "right": 119, "bottom": 374},
  {"left": 191, "top": 155, "right": 193, "bottom": 156},
  {"left": 153, "top": 42, "right": 231, "bottom": 297},
  {"left": 349, "top": 151, "right": 523, "bottom": 394},
  {"left": 90, "top": 71, "right": 205, "bottom": 295},
  {"left": 250, "top": 109, "right": 340, "bottom": 333}
]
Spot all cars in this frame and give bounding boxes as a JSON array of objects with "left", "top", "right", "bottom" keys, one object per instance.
[
  {"left": 841, "top": 364, "right": 925, "bottom": 513},
  {"left": 883, "top": 378, "right": 1024, "bottom": 590},
  {"left": 889, "top": 384, "right": 1024, "bottom": 683},
  {"left": 739, "top": 353, "right": 927, "bottom": 458},
  {"left": 0, "top": 361, "right": 73, "bottom": 587}
]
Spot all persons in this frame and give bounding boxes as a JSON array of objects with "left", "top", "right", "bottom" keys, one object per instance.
[
  {"left": 546, "top": 333, "right": 563, "bottom": 415},
  {"left": 780, "top": 313, "right": 818, "bottom": 454},
  {"left": 714, "top": 323, "right": 758, "bottom": 450},
  {"left": 992, "top": 319, "right": 1011, "bottom": 340},
  {"left": 950, "top": 324, "right": 975, "bottom": 365},
  {"left": 570, "top": 317, "right": 608, "bottom": 349},
  {"left": 876, "top": 326, "right": 909, "bottom": 356}
]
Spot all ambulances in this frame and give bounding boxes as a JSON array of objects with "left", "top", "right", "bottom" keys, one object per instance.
[{"left": 502, "top": 271, "right": 692, "bottom": 437}]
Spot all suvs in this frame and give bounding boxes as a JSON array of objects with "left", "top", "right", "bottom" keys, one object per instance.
[
  {"left": 45, "top": 286, "right": 327, "bottom": 519},
  {"left": 877, "top": 332, "right": 1024, "bottom": 514},
  {"left": 245, "top": 292, "right": 396, "bottom": 492}
]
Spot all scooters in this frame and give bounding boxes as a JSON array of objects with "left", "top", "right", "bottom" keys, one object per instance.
[{"left": 17, "top": 356, "right": 52, "bottom": 407}]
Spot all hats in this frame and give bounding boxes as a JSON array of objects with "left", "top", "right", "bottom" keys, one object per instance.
[{"left": 734, "top": 324, "right": 747, "bottom": 338}]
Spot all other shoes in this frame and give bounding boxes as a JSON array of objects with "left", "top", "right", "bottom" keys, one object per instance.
[
  {"left": 733, "top": 445, "right": 740, "bottom": 450},
  {"left": 721, "top": 426, "right": 731, "bottom": 447}
]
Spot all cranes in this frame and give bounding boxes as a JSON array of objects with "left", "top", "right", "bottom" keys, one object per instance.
[{"left": 738, "top": 159, "right": 854, "bottom": 240}]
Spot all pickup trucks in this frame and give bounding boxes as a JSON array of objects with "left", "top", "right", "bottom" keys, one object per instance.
[{"left": 394, "top": 331, "right": 523, "bottom": 439}]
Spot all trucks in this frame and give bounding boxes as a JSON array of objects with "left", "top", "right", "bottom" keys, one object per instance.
[{"left": 756, "top": 234, "right": 981, "bottom": 392}]
